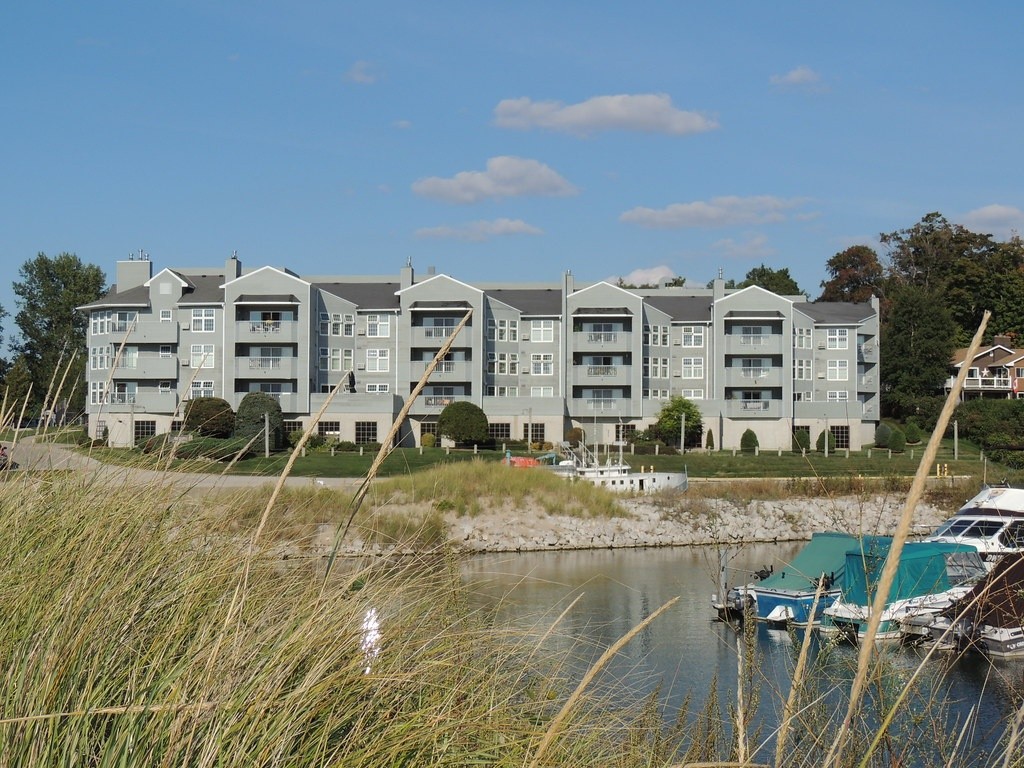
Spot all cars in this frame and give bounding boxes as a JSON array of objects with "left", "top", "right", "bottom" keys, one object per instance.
[{"left": 0, "top": 443, "right": 7, "bottom": 470}]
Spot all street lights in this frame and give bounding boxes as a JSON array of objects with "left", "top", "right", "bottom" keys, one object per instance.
[{"left": 260, "top": 413, "right": 269, "bottom": 458}]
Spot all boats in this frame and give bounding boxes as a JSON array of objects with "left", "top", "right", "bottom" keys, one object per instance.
[
  {"left": 909, "top": 488, "right": 1023, "bottom": 555},
  {"left": 502, "top": 394, "right": 688, "bottom": 493},
  {"left": 820, "top": 542, "right": 987, "bottom": 642},
  {"left": 726, "top": 533, "right": 894, "bottom": 627},
  {"left": 899, "top": 553, "right": 1024, "bottom": 658}
]
